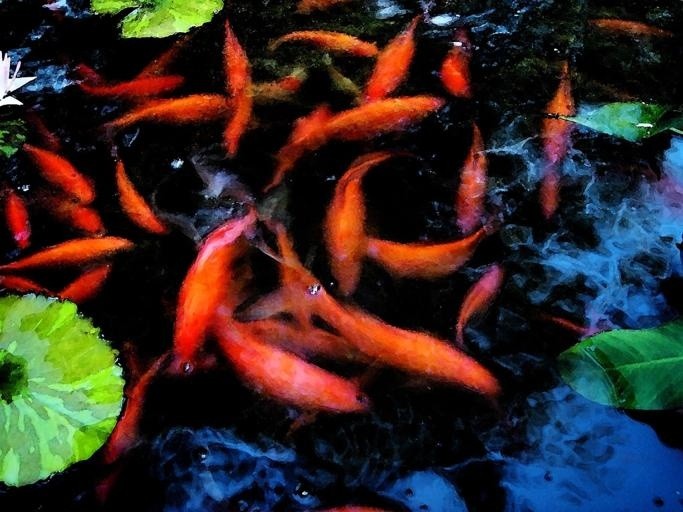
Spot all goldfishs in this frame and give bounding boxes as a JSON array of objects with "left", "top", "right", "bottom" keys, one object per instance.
[
  {"left": 0, "top": 2, "right": 583, "bottom": 467},
  {"left": 597, "top": 18, "right": 653, "bottom": 37}
]
